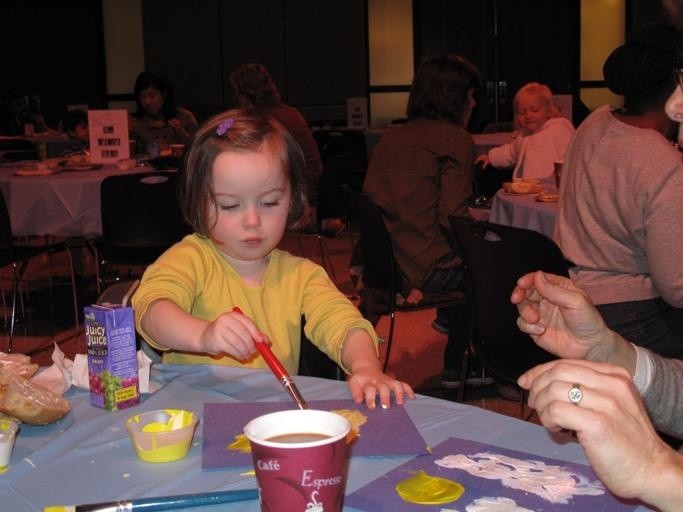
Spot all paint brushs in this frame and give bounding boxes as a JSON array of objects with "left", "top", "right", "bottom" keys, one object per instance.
[
  {"left": 234, "top": 306, "right": 309, "bottom": 410},
  {"left": 43, "top": 488, "right": 259, "bottom": 512}
]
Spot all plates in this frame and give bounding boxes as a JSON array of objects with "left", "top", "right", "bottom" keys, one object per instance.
[{"left": 502, "top": 175, "right": 560, "bottom": 203}]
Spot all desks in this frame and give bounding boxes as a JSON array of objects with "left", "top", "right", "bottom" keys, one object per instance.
[{"left": 0, "top": 361, "right": 650, "bottom": 512}]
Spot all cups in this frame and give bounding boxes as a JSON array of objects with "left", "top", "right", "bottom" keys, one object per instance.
[{"left": 242, "top": 407, "right": 352, "bottom": 512}]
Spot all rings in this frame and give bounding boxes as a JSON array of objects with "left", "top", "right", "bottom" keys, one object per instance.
[{"left": 567, "top": 384, "right": 582, "bottom": 406}]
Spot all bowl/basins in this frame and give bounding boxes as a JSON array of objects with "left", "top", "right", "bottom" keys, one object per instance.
[
  {"left": 126, "top": 407, "right": 200, "bottom": 462},
  {"left": 0, "top": 418, "right": 20, "bottom": 473}
]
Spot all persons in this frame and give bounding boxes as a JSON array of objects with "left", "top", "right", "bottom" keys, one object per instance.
[
  {"left": 126, "top": 109, "right": 416, "bottom": 410},
  {"left": 129, "top": 70, "right": 200, "bottom": 154},
  {"left": 227, "top": 62, "right": 322, "bottom": 209},
  {"left": 553, "top": 21, "right": 683, "bottom": 358},
  {"left": 511, "top": 43, "right": 683, "bottom": 512},
  {"left": 473, "top": 82, "right": 575, "bottom": 181},
  {"left": 59, "top": 110, "right": 88, "bottom": 158},
  {"left": 363, "top": 54, "right": 495, "bottom": 387}
]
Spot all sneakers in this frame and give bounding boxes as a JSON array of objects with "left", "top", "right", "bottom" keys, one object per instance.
[
  {"left": 429, "top": 314, "right": 450, "bottom": 334},
  {"left": 438, "top": 366, "right": 498, "bottom": 390}
]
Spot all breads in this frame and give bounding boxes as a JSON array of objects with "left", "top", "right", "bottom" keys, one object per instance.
[{"left": 0, "top": 352, "right": 71, "bottom": 426}]
